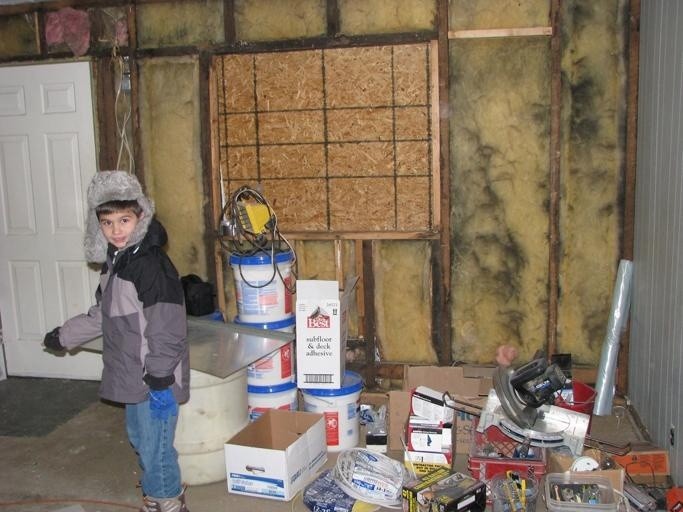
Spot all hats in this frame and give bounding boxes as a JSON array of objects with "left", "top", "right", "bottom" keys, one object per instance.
[{"left": 85, "top": 170, "right": 154, "bottom": 263}]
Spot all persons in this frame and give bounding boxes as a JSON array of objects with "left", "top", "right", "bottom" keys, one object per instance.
[{"left": 44, "top": 170, "right": 190, "bottom": 512}]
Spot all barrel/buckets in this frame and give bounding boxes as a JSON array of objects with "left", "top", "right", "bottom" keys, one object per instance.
[
  {"left": 247, "top": 379, "right": 299, "bottom": 425},
  {"left": 228, "top": 249, "right": 294, "bottom": 324},
  {"left": 233, "top": 314, "right": 297, "bottom": 388},
  {"left": 302, "top": 369, "right": 364, "bottom": 454}
]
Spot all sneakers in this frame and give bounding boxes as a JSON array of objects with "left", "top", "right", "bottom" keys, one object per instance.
[{"left": 138, "top": 484, "right": 189, "bottom": 512}]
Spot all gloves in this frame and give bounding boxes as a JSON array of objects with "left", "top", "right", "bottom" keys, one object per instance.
[
  {"left": 44, "top": 327, "right": 64, "bottom": 352},
  {"left": 148, "top": 387, "right": 177, "bottom": 421}
]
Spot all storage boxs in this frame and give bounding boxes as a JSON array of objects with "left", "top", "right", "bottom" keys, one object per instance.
[
  {"left": 295, "top": 274, "right": 358, "bottom": 388},
  {"left": 405, "top": 466, "right": 486, "bottom": 512},
  {"left": 360, "top": 359, "right": 597, "bottom": 465},
  {"left": 224, "top": 409, "right": 328, "bottom": 502},
  {"left": 545, "top": 473, "right": 614, "bottom": 512}
]
